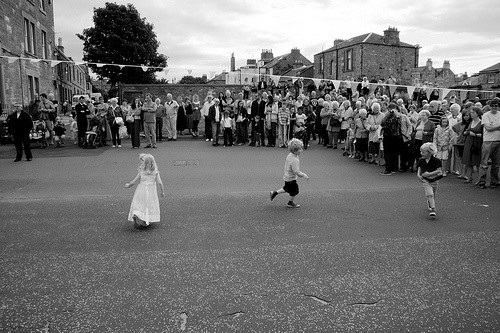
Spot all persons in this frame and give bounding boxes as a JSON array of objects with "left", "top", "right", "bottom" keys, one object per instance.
[
  {"left": 269, "top": 137, "right": 309, "bottom": 208},
  {"left": 125, "top": 152, "right": 166, "bottom": 229},
  {"left": 6, "top": 76, "right": 500, "bottom": 190},
  {"left": 416, "top": 142, "right": 443, "bottom": 217}
]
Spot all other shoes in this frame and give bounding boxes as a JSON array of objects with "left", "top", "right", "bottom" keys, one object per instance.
[
  {"left": 39, "top": 137, "right": 177, "bottom": 148},
  {"left": 14, "top": 159, "right": 22, "bottom": 162},
  {"left": 318, "top": 141, "right": 413, "bottom": 175},
  {"left": 429, "top": 211, "right": 437, "bottom": 216},
  {"left": 442, "top": 170, "right": 498, "bottom": 189},
  {"left": 427, "top": 200, "right": 432, "bottom": 211},
  {"left": 143, "top": 222, "right": 150, "bottom": 226},
  {"left": 287, "top": 202, "right": 300, "bottom": 208},
  {"left": 192, "top": 134, "right": 317, "bottom": 149},
  {"left": 270, "top": 191, "right": 278, "bottom": 201},
  {"left": 28, "top": 158, "right": 32, "bottom": 161},
  {"left": 133, "top": 216, "right": 139, "bottom": 228}
]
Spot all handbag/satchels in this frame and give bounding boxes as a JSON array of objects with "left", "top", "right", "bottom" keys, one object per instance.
[
  {"left": 119, "top": 126, "right": 129, "bottom": 139},
  {"left": 49, "top": 113, "right": 56, "bottom": 121},
  {"left": 126, "top": 116, "right": 134, "bottom": 123},
  {"left": 373, "top": 125, "right": 382, "bottom": 144},
  {"left": 341, "top": 121, "right": 349, "bottom": 131},
  {"left": 330, "top": 117, "right": 340, "bottom": 127},
  {"left": 114, "top": 117, "right": 124, "bottom": 126}
]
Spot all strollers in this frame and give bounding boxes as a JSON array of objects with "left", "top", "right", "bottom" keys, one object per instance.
[
  {"left": 83, "top": 116, "right": 106, "bottom": 149},
  {"left": 292, "top": 119, "right": 310, "bottom": 150},
  {"left": 29, "top": 119, "right": 51, "bottom": 149}
]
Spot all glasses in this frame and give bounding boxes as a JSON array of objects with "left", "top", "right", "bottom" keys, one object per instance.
[{"left": 14, "top": 106, "right": 19, "bottom": 107}]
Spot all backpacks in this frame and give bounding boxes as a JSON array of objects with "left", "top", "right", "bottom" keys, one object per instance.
[{"left": 383, "top": 114, "right": 398, "bottom": 134}]
